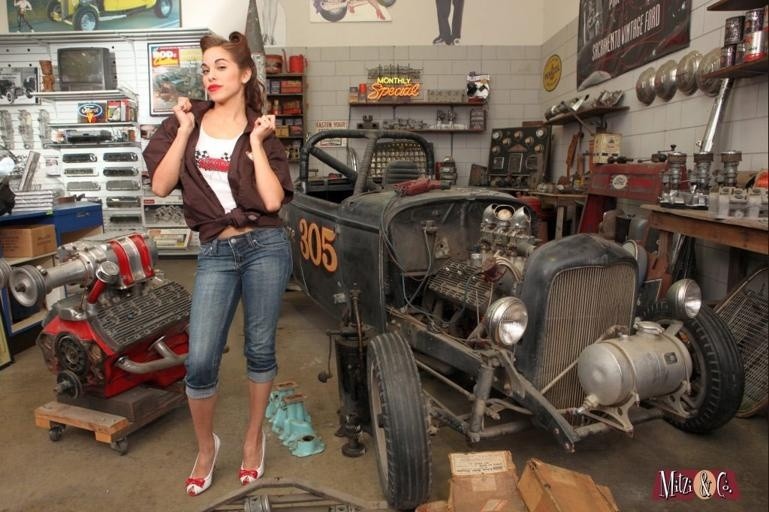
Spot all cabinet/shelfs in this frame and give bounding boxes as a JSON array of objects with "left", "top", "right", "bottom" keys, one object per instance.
[
  {"left": 704, "top": 0, "right": 769, "bottom": 79},
  {"left": 0, "top": 204, "right": 103, "bottom": 356},
  {"left": 350, "top": 102, "right": 487, "bottom": 134},
  {"left": 264, "top": 72, "right": 308, "bottom": 160},
  {"left": 31, "top": 88, "right": 200, "bottom": 257}
]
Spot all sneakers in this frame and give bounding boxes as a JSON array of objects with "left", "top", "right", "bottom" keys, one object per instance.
[{"left": 433, "top": 36, "right": 447, "bottom": 44}]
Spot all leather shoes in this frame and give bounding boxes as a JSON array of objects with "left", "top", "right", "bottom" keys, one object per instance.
[
  {"left": 187, "top": 432, "right": 221, "bottom": 496},
  {"left": 240, "top": 431, "right": 266, "bottom": 485}
]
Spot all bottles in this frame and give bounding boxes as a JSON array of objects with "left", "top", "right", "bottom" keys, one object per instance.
[
  {"left": 348, "top": 81, "right": 367, "bottom": 105},
  {"left": 270, "top": 98, "right": 281, "bottom": 115}
]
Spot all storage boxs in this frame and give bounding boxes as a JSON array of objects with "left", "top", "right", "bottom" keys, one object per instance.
[
  {"left": 416, "top": 450, "right": 620, "bottom": 512},
  {"left": 0, "top": 224, "right": 58, "bottom": 258}
]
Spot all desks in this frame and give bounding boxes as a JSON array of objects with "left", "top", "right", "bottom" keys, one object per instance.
[
  {"left": 529, "top": 192, "right": 587, "bottom": 235},
  {"left": 640, "top": 204, "right": 769, "bottom": 275}
]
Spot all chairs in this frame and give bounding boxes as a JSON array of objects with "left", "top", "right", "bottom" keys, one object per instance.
[{"left": 381, "top": 160, "right": 420, "bottom": 189}]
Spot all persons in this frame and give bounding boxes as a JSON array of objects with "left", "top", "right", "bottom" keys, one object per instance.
[
  {"left": 433, "top": 0, "right": 463, "bottom": 44},
  {"left": 142, "top": 30, "right": 295, "bottom": 496}
]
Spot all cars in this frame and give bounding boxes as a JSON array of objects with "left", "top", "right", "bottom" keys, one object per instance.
[
  {"left": 288, "top": 125, "right": 747, "bottom": 512},
  {"left": 48, "top": 0, "right": 173, "bottom": 31}
]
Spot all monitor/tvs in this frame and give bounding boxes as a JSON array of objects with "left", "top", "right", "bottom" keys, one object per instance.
[{"left": 57, "top": 47, "right": 111, "bottom": 91}]
